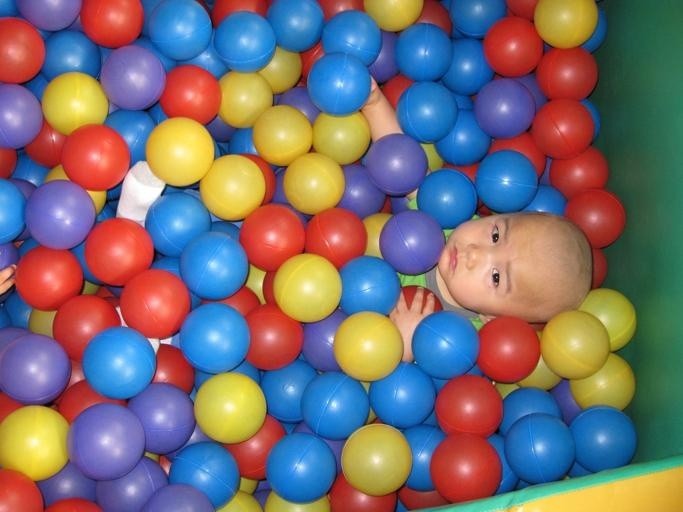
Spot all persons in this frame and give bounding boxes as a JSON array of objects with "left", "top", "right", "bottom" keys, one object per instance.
[{"left": 0, "top": 75, "right": 591, "bottom": 423}]
